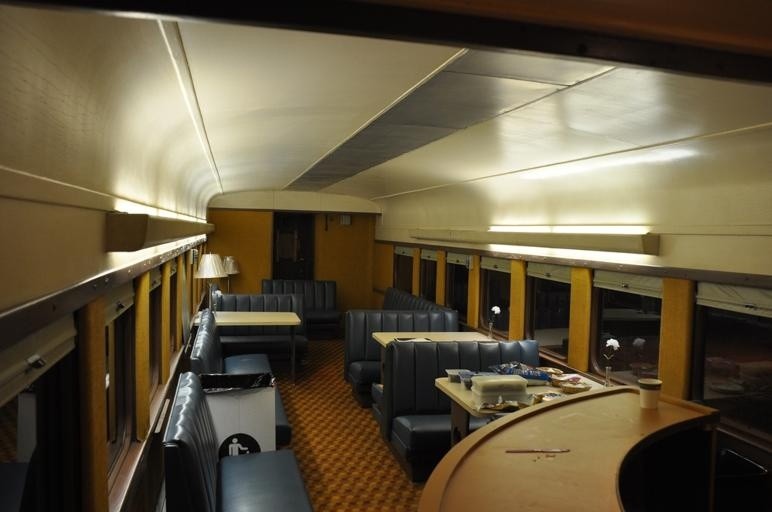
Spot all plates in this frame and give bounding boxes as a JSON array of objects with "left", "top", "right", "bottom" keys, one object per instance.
[{"left": 549, "top": 374, "right": 593, "bottom": 393}]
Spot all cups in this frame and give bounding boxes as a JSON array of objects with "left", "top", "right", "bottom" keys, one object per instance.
[{"left": 636, "top": 378, "right": 663, "bottom": 410}]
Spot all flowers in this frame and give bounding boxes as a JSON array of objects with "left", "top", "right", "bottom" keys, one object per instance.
[
  {"left": 633, "top": 338, "right": 646, "bottom": 350},
  {"left": 604, "top": 339, "right": 620, "bottom": 367}
]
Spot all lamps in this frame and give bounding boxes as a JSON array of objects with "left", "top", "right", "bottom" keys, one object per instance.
[
  {"left": 102, "top": 208, "right": 216, "bottom": 252},
  {"left": 407, "top": 228, "right": 659, "bottom": 256},
  {"left": 193, "top": 253, "right": 241, "bottom": 295}
]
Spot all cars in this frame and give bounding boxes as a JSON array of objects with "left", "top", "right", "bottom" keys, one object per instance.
[{"left": 533, "top": 303, "right": 659, "bottom": 353}]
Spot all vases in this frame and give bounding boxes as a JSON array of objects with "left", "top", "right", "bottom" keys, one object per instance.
[{"left": 604, "top": 366, "right": 612, "bottom": 386}]
